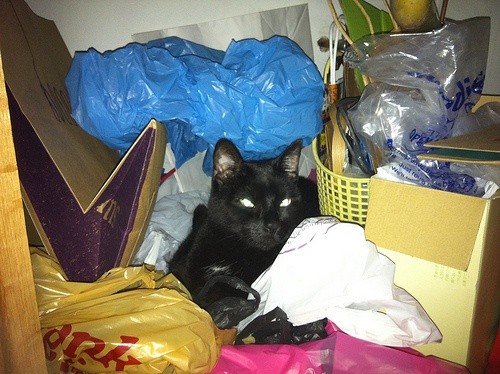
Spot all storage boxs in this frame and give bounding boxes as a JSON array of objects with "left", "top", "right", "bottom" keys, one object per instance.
[{"left": 362, "top": 175, "right": 500, "bottom": 374}]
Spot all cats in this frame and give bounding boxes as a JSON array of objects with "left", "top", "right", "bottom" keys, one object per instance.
[{"left": 167, "top": 137, "right": 322, "bottom": 302}]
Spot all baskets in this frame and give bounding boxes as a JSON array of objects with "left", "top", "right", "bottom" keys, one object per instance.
[{"left": 312, "top": 124, "right": 372, "bottom": 228}]
